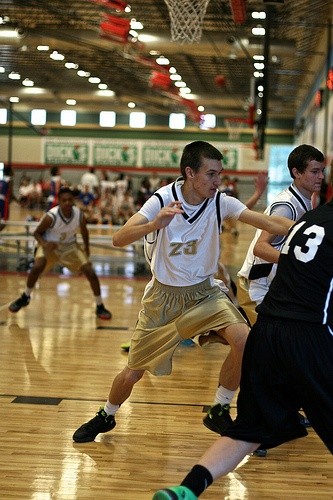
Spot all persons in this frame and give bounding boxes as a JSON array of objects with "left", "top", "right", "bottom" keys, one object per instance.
[
  {"left": 9, "top": 188, "right": 113, "bottom": 321},
  {"left": 72, "top": 141, "right": 297, "bottom": 444},
  {"left": 237, "top": 144, "right": 326, "bottom": 327},
  {"left": 120, "top": 169, "right": 271, "bottom": 352},
  {"left": 0, "top": 164, "right": 160, "bottom": 235},
  {"left": 151, "top": 191, "right": 333, "bottom": 500}
]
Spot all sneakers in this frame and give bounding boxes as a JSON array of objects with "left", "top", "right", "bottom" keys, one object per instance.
[
  {"left": 95, "top": 303, "right": 112, "bottom": 319},
  {"left": 72, "top": 407, "right": 116, "bottom": 443},
  {"left": 202, "top": 403, "right": 232, "bottom": 434},
  {"left": 7, "top": 292, "right": 30, "bottom": 312},
  {"left": 152, "top": 485, "right": 197, "bottom": 500}
]
